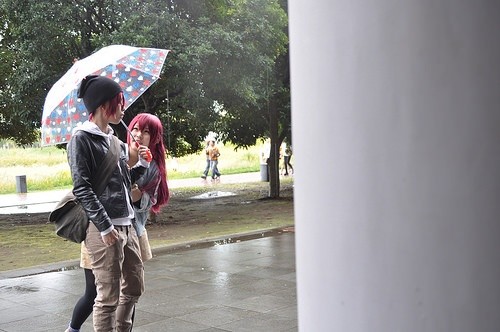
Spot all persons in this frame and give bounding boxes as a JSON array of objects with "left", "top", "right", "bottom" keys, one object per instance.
[
  {"left": 66, "top": 111, "right": 169, "bottom": 332},
  {"left": 201, "top": 141, "right": 221, "bottom": 181},
  {"left": 259, "top": 137, "right": 293, "bottom": 176},
  {"left": 66, "top": 74, "right": 152, "bottom": 332}
]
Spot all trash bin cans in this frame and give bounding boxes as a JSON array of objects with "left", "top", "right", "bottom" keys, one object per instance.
[
  {"left": 260, "top": 162, "right": 269, "bottom": 182},
  {"left": 16, "top": 174, "right": 27, "bottom": 193}
]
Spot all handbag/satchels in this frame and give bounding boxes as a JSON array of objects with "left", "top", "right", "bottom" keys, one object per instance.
[{"left": 49, "top": 193, "right": 90, "bottom": 244}]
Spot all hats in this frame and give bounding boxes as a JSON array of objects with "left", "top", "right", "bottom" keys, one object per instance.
[{"left": 76, "top": 74, "right": 124, "bottom": 115}]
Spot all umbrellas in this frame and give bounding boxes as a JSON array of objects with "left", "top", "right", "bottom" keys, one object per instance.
[{"left": 41, "top": 45, "right": 171, "bottom": 162}]
